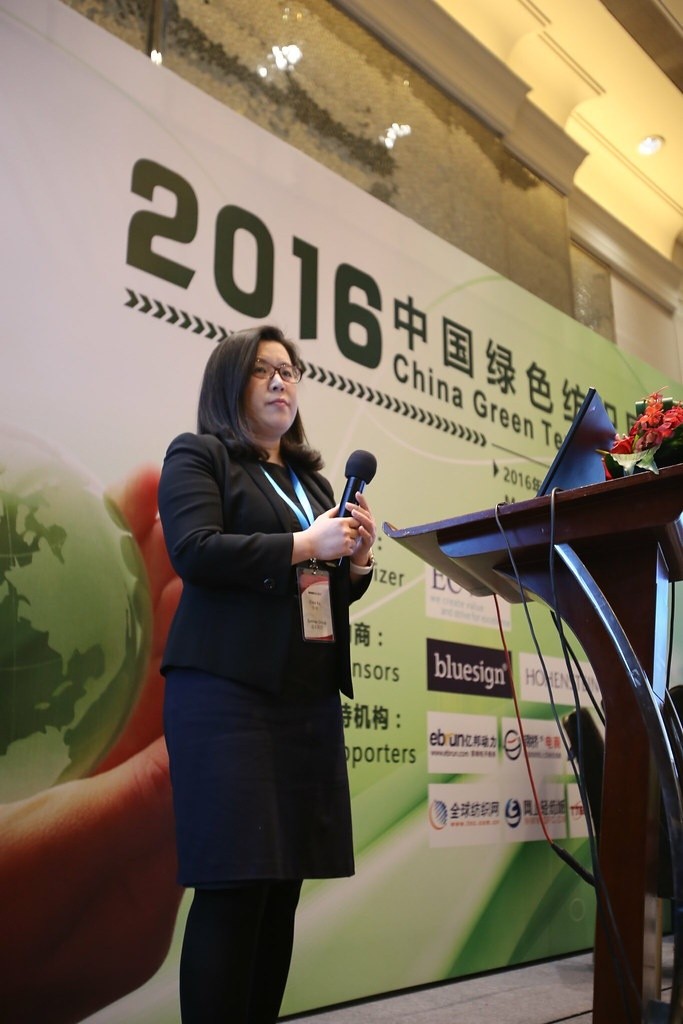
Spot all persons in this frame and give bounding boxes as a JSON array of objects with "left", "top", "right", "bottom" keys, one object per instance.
[
  {"left": 0, "top": 460, "right": 186, "bottom": 1024},
  {"left": 156, "top": 325, "right": 376, "bottom": 1024}
]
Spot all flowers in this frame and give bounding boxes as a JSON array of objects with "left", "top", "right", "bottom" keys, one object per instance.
[{"left": 592, "top": 387, "right": 683, "bottom": 482}]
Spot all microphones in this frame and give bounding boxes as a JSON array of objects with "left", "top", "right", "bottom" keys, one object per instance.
[{"left": 323, "top": 450, "right": 377, "bottom": 568}]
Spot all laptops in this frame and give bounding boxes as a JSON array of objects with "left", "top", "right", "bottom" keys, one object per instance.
[{"left": 534, "top": 387, "right": 618, "bottom": 496}]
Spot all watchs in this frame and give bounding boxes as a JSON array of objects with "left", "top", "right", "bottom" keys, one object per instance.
[{"left": 350, "top": 548, "right": 375, "bottom": 575}]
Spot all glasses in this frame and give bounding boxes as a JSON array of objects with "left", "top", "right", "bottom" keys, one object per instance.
[{"left": 250, "top": 357, "right": 303, "bottom": 384}]
